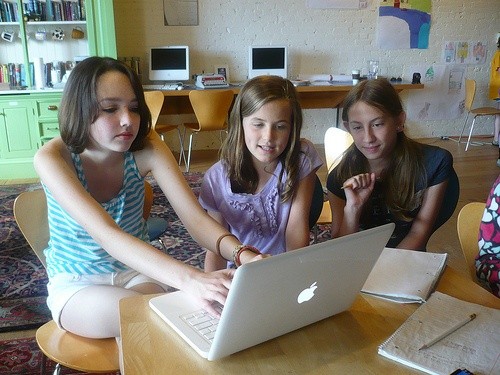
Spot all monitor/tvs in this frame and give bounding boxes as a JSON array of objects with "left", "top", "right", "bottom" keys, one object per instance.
[
  {"left": 249, "top": 46, "right": 288, "bottom": 79},
  {"left": 149, "top": 46, "right": 190, "bottom": 84}
]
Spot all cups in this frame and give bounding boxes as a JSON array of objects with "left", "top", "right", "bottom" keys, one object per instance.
[
  {"left": 351, "top": 69, "right": 360, "bottom": 87},
  {"left": 35, "top": 26, "right": 47, "bottom": 41},
  {"left": 1, "top": 28, "right": 17, "bottom": 43},
  {"left": 71, "top": 26, "right": 83, "bottom": 39},
  {"left": 17, "top": 31, "right": 31, "bottom": 40},
  {"left": 51, "top": 25, "right": 65, "bottom": 40},
  {"left": 367, "top": 64, "right": 378, "bottom": 81}
]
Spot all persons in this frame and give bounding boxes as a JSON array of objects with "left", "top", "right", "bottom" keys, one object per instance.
[
  {"left": 476, "top": 179, "right": 500, "bottom": 299},
  {"left": 33, "top": 56, "right": 271, "bottom": 375},
  {"left": 488, "top": 37, "right": 500, "bottom": 148},
  {"left": 326, "top": 77, "right": 454, "bottom": 251},
  {"left": 198, "top": 75, "right": 323, "bottom": 273}
]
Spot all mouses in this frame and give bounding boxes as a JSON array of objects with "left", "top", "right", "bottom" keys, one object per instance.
[{"left": 178, "top": 85, "right": 184, "bottom": 90}]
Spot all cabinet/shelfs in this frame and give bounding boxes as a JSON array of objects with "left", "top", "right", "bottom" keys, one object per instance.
[{"left": 0, "top": 0, "right": 118, "bottom": 183}]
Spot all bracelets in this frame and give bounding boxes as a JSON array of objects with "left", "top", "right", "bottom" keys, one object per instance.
[
  {"left": 216, "top": 233, "right": 240, "bottom": 262},
  {"left": 232, "top": 244, "right": 261, "bottom": 267}
]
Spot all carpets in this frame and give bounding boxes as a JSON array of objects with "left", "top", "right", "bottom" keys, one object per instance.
[
  {"left": 0, "top": 170, "right": 338, "bottom": 333},
  {"left": 0, "top": 336, "right": 120, "bottom": 375}
]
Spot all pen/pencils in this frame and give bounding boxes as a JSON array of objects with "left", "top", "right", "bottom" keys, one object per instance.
[
  {"left": 419, "top": 314, "right": 476, "bottom": 350},
  {"left": 341, "top": 184, "right": 353, "bottom": 189}
]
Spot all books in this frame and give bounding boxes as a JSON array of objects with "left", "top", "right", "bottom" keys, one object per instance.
[
  {"left": 378, "top": 290, "right": 500, "bottom": 375},
  {"left": 0, "top": 0, "right": 86, "bottom": 89}
]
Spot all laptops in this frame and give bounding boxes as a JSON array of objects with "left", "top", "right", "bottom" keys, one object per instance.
[{"left": 150, "top": 222, "right": 396, "bottom": 361}]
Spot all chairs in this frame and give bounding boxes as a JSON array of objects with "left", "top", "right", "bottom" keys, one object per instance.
[
  {"left": 13, "top": 180, "right": 139, "bottom": 375},
  {"left": 457, "top": 200, "right": 483, "bottom": 284},
  {"left": 324, "top": 126, "right": 354, "bottom": 172},
  {"left": 142, "top": 90, "right": 188, "bottom": 174},
  {"left": 180, "top": 90, "right": 232, "bottom": 174},
  {"left": 458, "top": 78, "right": 500, "bottom": 151}
]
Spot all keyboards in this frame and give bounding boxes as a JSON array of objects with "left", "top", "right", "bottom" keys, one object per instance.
[{"left": 142, "top": 84, "right": 178, "bottom": 90}]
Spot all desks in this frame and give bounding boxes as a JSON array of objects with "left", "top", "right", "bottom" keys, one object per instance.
[
  {"left": 118, "top": 260, "right": 500, "bottom": 375},
  {"left": 143, "top": 82, "right": 424, "bottom": 115}
]
